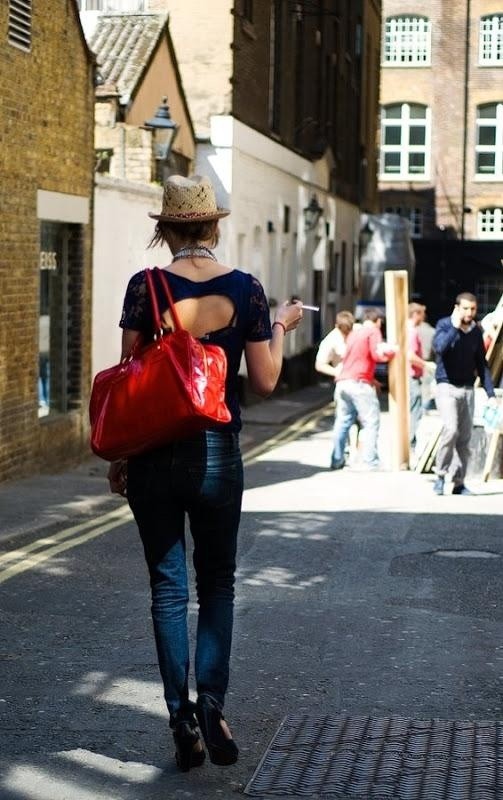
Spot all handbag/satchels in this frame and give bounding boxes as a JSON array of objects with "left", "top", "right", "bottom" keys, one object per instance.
[{"left": 88, "top": 327, "right": 235, "bottom": 463}]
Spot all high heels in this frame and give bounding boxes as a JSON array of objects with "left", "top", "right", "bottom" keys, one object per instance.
[
  {"left": 172, "top": 720, "right": 206, "bottom": 772},
  {"left": 196, "top": 694, "right": 239, "bottom": 767}
]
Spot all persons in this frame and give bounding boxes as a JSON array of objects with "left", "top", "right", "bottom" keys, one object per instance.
[
  {"left": 104, "top": 173, "right": 304, "bottom": 773},
  {"left": 313, "top": 293, "right": 497, "bottom": 497}
]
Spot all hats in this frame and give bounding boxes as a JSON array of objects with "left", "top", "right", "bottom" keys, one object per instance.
[{"left": 146, "top": 173, "right": 230, "bottom": 224}]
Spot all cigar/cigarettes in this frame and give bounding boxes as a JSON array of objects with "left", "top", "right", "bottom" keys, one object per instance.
[{"left": 300, "top": 302, "right": 320, "bottom": 313}]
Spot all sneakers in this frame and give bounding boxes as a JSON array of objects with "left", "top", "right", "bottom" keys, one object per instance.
[
  {"left": 433, "top": 478, "right": 446, "bottom": 495},
  {"left": 451, "top": 484, "right": 475, "bottom": 497}
]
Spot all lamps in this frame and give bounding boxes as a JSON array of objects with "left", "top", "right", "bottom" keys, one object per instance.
[
  {"left": 303, "top": 194, "right": 321, "bottom": 233},
  {"left": 143, "top": 96, "right": 177, "bottom": 183}
]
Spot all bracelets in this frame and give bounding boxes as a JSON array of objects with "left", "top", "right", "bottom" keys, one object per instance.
[{"left": 271, "top": 320, "right": 287, "bottom": 338}]
